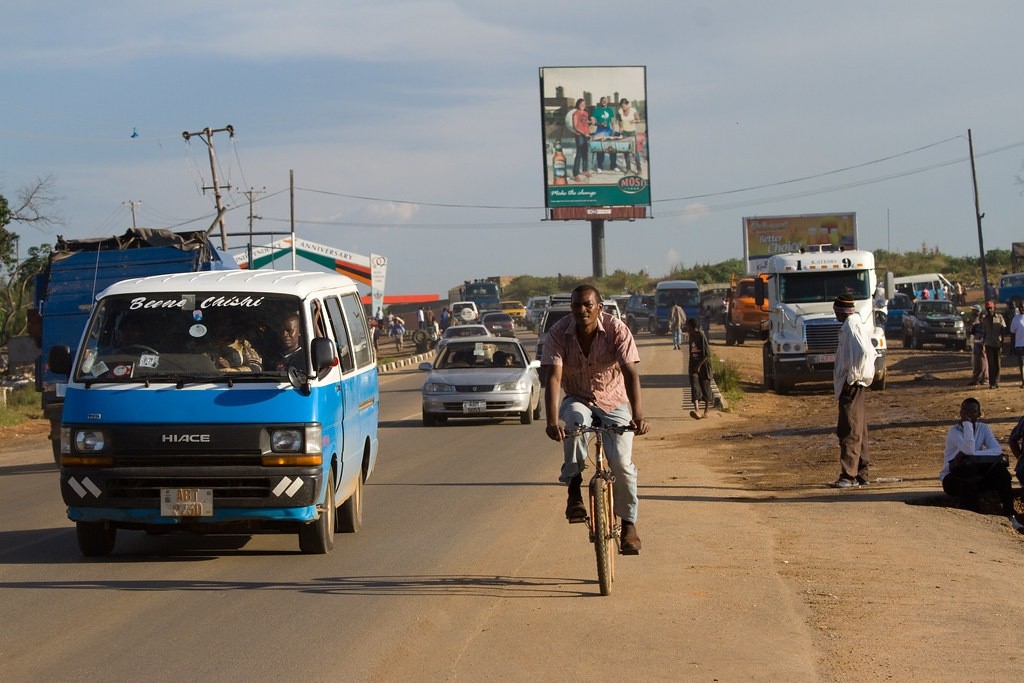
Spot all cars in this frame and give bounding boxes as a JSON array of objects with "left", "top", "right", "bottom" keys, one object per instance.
[
  {"left": 700, "top": 287, "right": 729, "bottom": 311},
  {"left": 884, "top": 294, "right": 916, "bottom": 332},
  {"left": 438, "top": 324, "right": 500, "bottom": 362},
  {"left": 419, "top": 337, "right": 543, "bottom": 427},
  {"left": 482, "top": 313, "right": 516, "bottom": 338}
]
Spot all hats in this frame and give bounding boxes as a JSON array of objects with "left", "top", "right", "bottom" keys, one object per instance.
[
  {"left": 833, "top": 295, "right": 855, "bottom": 313},
  {"left": 969, "top": 304, "right": 982, "bottom": 312}
]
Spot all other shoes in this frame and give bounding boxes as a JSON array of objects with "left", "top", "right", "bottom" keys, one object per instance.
[
  {"left": 573, "top": 175, "right": 581, "bottom": 182},
  {"left": 690, "top": 411, "right": 701, "bottom": 419},
  {"left": 980, "top": 379, "right": 987, "bottom": 384},
  {"left": 1011, "top": 514, "right": 1023, "bottom": 529},
  {"left": 598, "top": 169, "right": 603, "bottom": 173},
  {"left": 990, "top": 385, "right": 996, "bottom": 389},
  {"left": 996, "top": 385, "right": 1000, "bottom": 389},
  {"left": 967, "top": 381, "right": 980, "bottom": 385},
  {"left": 610, "top": 167, "right": 620, "bottom": 171},
  {"left": 857, "top": 478, "right": 869, "bottom": 485},
  {"left": 829, "top": 479, "right": 859, "bottom": 487},
  {"left": 583, "top": 172, "right": 591, "bottom": 177},
  {"left": 701, "top": 414, "right": 707, "bottom": 417}
]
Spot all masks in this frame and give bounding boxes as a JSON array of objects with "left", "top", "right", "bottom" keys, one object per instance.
[{"left": 985, "top": 302, "right": 995, "bottom": 307}]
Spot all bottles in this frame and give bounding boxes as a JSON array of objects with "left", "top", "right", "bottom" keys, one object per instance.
[{"left": 82, "top": 347, "right": 98, "bottom": 373}]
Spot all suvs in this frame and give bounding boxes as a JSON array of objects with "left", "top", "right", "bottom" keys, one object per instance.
[
  {"left": 525, "top": 296, "right": 548, "bottom": 331},
  {"left": 625, "top": 294, "right": 655, "bottom": 336},
  {"left": 447, "top": 301, "right": 479, "bottom": 324},
  {"left": 998, "top": 272, "right": 1024, "bottom": 306},
  {"left": 900, "top": 299, "right": 968, "bottom": 352},
  {"left": 536, "top": 294, "right": 632, "bottom": 374},
  {"left": 879, "top": 273, "right": 956, "bottom": 303},
  {"left": 500, "top": 301, "right": 527, "bottom": 327}
]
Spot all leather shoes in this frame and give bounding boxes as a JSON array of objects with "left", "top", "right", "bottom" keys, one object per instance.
[
  {"left": 565, "top": 496, "right": 587, "bottom": 518},
  {"left": 620, "top": 525, "right": 641, "bottom": 550}
]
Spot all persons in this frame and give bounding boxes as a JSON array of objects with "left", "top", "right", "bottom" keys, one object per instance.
[
  {"left": 369, "top": 278, "right": 523, "bottom": 369},
  {"left": 568, "top": 97, "right": 642, "bottom": 182},
  {"left": 939, "top": 397, "right": 1024, "bottom": 537},
  {"left": 969, "top": 298, "right": 1024, "bottom": 390},
  {"left": 684, "top": 317, "right": 713, "bottom": 420},
  {"left": 541, "top": 286, "right": 650, "bottom": 552},
  {"left": 100, "top": 298, "right": 327, "bottom": 380},
  {"left": 667, "top": 300, "right": 687, "bottom": 350},
  {"left": 828, "top": 294, "right": 878, "bottom": 488},
  {"left": 894, "top": 268, "right": 1011, "bottom": 314},
  {"left": 697, "top": 297, "right": 730, "bottom": 345}
]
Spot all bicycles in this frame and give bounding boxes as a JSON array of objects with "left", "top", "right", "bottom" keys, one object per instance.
[{"left": 554, "top": 412, "right": 640, "bottom": 597}]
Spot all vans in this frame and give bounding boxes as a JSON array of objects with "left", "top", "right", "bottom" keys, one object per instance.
[
  {"left": 655, "top": 281, "right": 704, "bottom": 335},
  {"left": 49, "top": 263, "right": 381, "bottom": 557}
]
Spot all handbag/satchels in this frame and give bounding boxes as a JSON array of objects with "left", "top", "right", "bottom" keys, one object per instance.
[
  {"left": 948, "top": 452, "right": 1010, "bottom": 472},
  {"left": 671, "top": 321, "right": 678, "bottom": 330}
]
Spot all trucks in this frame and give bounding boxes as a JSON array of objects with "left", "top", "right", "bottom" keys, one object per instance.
[
  {"left": 753, "top": 246, "right": 889, "bottom": 394},
  {"left": 724, "top": 271, "right": 772, "bottom": 345},
  {"left": 448, "top": 278, "right": 503, "bottom": 312},
  {"left": 26, "top": 242, "right": 243, "bottom": 465}
]
